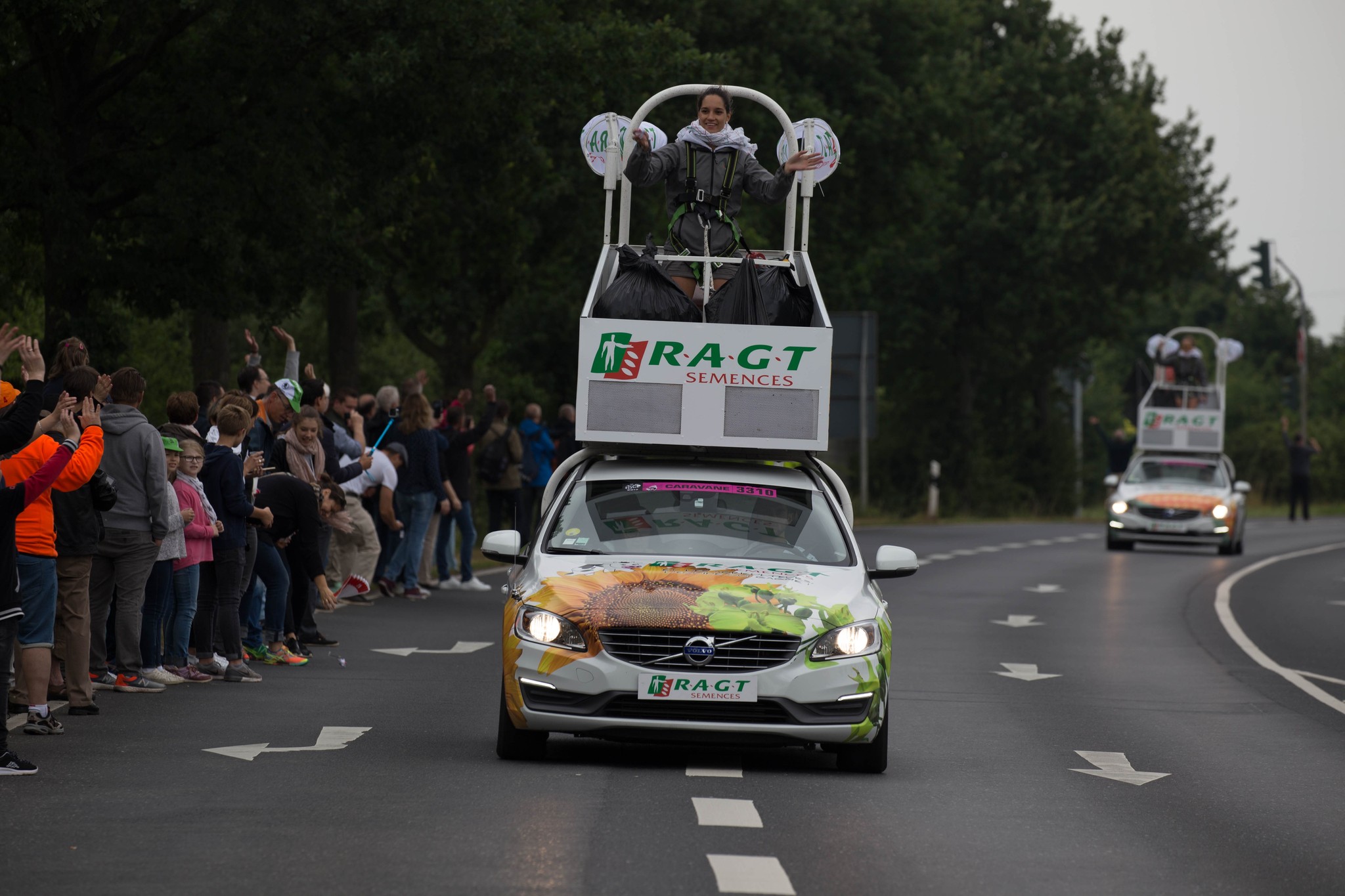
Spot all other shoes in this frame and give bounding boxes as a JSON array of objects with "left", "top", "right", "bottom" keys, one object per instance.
[
  {"left": 8, "top": 702, "right": 51, "bottom": 714},
  {"left": 68, "top": 702, "right": 99, "bottom": 715}
]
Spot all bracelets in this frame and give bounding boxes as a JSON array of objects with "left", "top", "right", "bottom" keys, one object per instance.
[{"left": 37, "top": 420, "right": 46, "bottom": 434}]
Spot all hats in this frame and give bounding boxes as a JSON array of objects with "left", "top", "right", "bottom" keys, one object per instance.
[
  {"left": 387, "top": 442, "right": 409, "bottom": 469},
  {"left": 160, "top": 436, "right": 185, "bottom": 452},
  {"left": 274, "top": 378, "right": 302, "bottom": 413},
  {"left": 0, "top": 380, "right": 22, "bottom": 408}
]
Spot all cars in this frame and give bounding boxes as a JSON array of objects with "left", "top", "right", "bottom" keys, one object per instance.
[
  {"left": 480, "top": 454, "right": 920, "bottom": 773},
  {"left": 1102, "top": 456, "right": 1251, "bottom": 555}
]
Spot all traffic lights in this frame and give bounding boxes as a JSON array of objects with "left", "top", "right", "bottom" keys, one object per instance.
[{"left": 1249, "top": 241, "right": 1270, "bottom": 288}]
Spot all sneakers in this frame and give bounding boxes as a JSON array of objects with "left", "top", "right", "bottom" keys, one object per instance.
[
  {"left": 317, "top": 573, "right": 492, "bottom": 612},
  {"left": 240, "top": 642, "right": 250, "bottom": 664},
  {"left": 263, "top": 645, "right": 309, "bottom": 667},
  {"left": 141, "top": 667, "right": 186, "bottom": 684},
  {"left": 164, "top": 664, "right": 212, "bottom": 683},
  {"left": 298, "top": 632, "right": 340, "bottom": 646},
  {"left": 188, "top": 655, "right": 199, "bottom": 666},
  {"left": 89, "top": 670, "right": 117, "bottom": 690},
  {"left": 243, "top": 646, "right": 268, "bottom": 661},
  {"left": 195, "top": 661, "right": 226, "bottom": 680},
  {"left": 114, "top": 674, "right": 167, "bottom": 693},
  {"left": 284, "top": 637, "right": 314, "bottom": 658},
  {"left": 0, "top": 750, "right": 38, "bottom": 776},
  {"left": 23, "top": 709, "right": 64, "bottom": 734},
  {"left": 223, "top": 663, "right": 263, "bottom": 683},
  {"left": 212, "top": 652, "right": 229, "bottom": 671}
]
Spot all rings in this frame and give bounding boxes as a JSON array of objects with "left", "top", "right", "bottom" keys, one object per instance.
[
  {"left": 28, "top": 349, "right": 34, "bottom": 351},
  {"left": 259, "top": 459, "right": 263, "bottom": 464},
  {"left": 90, "top": 410, "right": 95, "bottom": 412}
]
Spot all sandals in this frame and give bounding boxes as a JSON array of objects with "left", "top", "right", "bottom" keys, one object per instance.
[{"left": 47, "top": 684, "right": 97, "bottom": 701}]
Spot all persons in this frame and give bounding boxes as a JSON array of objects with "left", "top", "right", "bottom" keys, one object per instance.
[
  {"left": 375, "top": 392, "right": 463, "bottom": 600},
  {"left": 1123, "top": 357, "right": 1154, "bottom": 427},
  {"left": 631, "top": 87, "right": 825, "bottom": 306},
  {"left": 1088, "top": 415, "right": 1138, "bottom": 499},
  {"left": 1155, "top": 335, "right": 1209, "bottom": 410},
  {"left": 0, "top": 323, "right": 584, "bottom": 776},
  {"left": 194, "top": 404, "right": 274, "bottom": 681},
  {"left": 1284, "top": 433, "right": 1323, "bottom": 521},
  {"left": 89, "top": 366, "right": 169, "bottom": 693}
]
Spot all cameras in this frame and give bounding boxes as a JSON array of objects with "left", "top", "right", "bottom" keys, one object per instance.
[{"left": 390, "top": 408, "right": 400, "bottom": 419}]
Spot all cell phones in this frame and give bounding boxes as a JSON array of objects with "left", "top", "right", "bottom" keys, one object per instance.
[
  {"left": 106, "top": 475, "right": 117, "bottom": 486},
  {"left": 248, "top": 466, "right": 277, "bottom": 472}
]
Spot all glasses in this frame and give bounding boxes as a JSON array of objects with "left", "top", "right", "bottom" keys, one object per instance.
[
  {"left": 181, "top": 455, "right": 205, "bottom": 462},
  {"left": 276, "top": 392, "right": 295, "bottom": 415}
]
